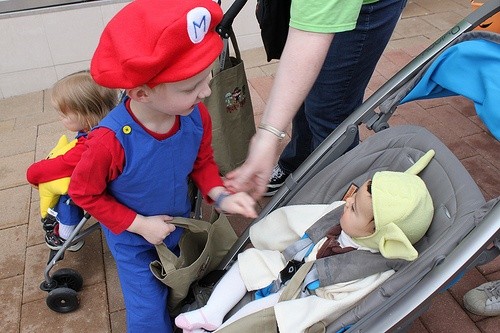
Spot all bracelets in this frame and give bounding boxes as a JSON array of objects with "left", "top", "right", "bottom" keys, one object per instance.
[
  {"left": 257, "top": 122, "right": 287, "bottom": 143},
  {"left": 213, "top": 189, "right": 235, "bottom": 208}
]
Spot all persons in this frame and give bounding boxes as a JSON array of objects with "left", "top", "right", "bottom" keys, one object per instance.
[
  {"left": 68, "top": 0, "right": 260, "bottom": 333},
  {"left": 462, "top": 280, "right": 500, "bottom": 317},
  {"left": 223, "top": 0, "right": 408, "bottom": 203},
  {"left": 173, "top": 149, "right": 442, "bottom": 333},
  {"left": 26, "top": 69, "right": 122, "bottom": 253}
]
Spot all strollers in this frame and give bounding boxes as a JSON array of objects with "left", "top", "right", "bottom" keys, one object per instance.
[
  {"left": 38, "top": 0, "right": 253, "bottom": 315},
  {"left": 170, "top": 1, "right": 500, "bottom": 333}
]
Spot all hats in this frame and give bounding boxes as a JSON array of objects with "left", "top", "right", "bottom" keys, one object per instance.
[
  {"left": 88, "top": 0, "right": 224, "bottom": 88},
  {"left": 351, "top": 147, "right": 434, "bottom": 261}
]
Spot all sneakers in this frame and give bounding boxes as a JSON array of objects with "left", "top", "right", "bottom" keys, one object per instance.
[
  {"left": 261, "top": 163, "right": 290, "bottom": 195},
  {"left": 463, "top": 278, "right": 500, "bottom": 316},
  {"left": 41, "top": 216, "right": 58, "bottom": 231},
  {"left": 45, "top": 231, "right": 85, "bottom": 252}
]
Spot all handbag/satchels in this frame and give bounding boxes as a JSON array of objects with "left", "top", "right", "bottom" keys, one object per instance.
[
  {"left": 148, "top": 205, "right": 239, "bottom": 311},
  {"left": 201, "top": 31, "right": 256, "bottom": 177}
]
